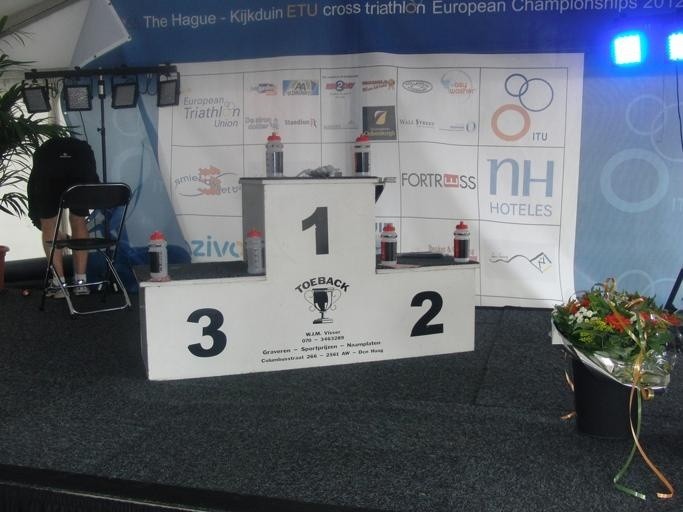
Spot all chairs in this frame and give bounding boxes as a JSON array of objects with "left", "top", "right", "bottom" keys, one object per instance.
[{"left": 40, "top": 182, "right": 135, "bottom": 320}]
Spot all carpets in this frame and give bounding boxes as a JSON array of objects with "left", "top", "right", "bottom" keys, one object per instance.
[{"left": 0, "top": 271, "right": 683, "bottom": 512}]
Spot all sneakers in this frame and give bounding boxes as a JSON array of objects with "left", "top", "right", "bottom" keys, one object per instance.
[
  {"left": 41, "top": 278, "right": 70, "bottom": 301},
  {"left": 71, "top": 277, "right": 91, "bottom": 297}
]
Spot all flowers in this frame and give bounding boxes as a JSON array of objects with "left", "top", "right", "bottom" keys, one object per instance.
[{"left": 550, "top": 276, "right": 683, "bottom": 500}]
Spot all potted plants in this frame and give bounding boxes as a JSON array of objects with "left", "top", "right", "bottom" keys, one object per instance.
[{"left": 0, "top": 16, "right": 83, "bottom": 293}]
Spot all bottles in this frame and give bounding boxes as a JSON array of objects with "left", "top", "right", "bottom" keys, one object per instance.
[
  {"left": 246, "top": 227, "right": 265, "bottom": 275},
  {"left": 354, "top": 133, "right": 370, "bottom": 177},
  {"left": 265, "top": 132, "right": 283, "bottom": 178},
  {"left": 148, "top": 232, "right": 168, "bottom": 279},
  {"left": 454, "top": 221, "right": 470, "bottom": 264},
  {"left": 380, "top": 224, "right": 397, "bottom": 267}
]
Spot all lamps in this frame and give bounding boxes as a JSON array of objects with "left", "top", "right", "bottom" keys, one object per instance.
[{"left": 20, "top": 62, "right": 180, "bottom": 114}]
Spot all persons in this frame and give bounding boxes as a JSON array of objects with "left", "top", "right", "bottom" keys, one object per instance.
[{"left": 26, "top": 136, "right": 101, "bottom": 300}]
